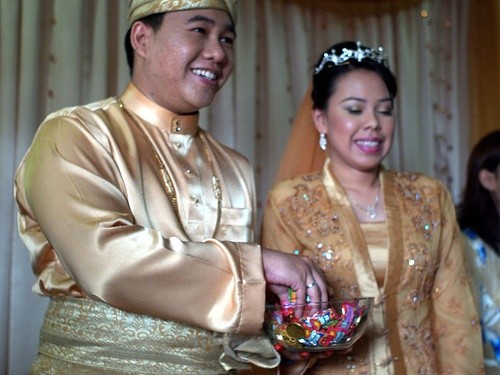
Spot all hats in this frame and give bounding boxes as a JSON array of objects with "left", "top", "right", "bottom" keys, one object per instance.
[{"left": 127, "top": 0, "right": 230, "bottom": 27}]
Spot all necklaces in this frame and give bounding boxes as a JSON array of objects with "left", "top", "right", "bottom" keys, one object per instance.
[{"left": 346, "top": 189, "right": 381, "bottom": 219}]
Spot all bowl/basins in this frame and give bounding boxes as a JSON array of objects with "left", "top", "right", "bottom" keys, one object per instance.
[{"left": 264, "top": 297, "right": 375, "bottom": 353}]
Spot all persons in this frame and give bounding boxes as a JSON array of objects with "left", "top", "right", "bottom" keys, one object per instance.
[
  {"left": 10, "top": 0, "right": 331, "bottom": 375},
  {"left": 453, "top": 127, "right": 500, "bottom": 375},
  {"left": 255, "top": 40, "right": 485, "bottom": 375}
]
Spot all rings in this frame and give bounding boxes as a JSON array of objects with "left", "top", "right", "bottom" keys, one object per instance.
[{"left": 306, "top": 280, "right": 316, "bottom": 288}]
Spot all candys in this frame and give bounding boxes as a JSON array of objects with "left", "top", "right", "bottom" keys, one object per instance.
[{"left": 263, "top": 287, "right": 366, "bottom": 350}]
